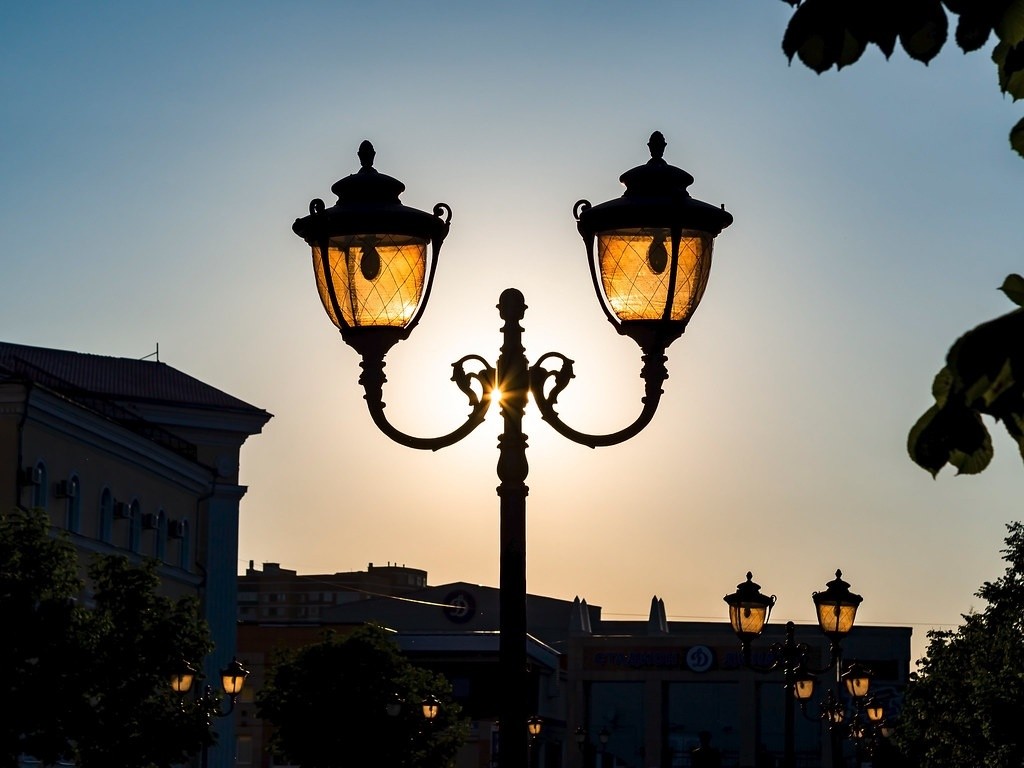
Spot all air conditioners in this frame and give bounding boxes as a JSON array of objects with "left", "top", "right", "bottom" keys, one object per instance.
[
  {"left": 27, "top": 467, "right": 42, "bottom": 486},
  {"left": 62, "top": 480, "right": 76, "bottom": 497},
  {"left": 145, "top": 513, "right": 158, "bottom": 528},
  {"left": 118, "top": 502, "right": 131, "bottom": 518},
  {"left": 172, "top": 520, "right": 185, "bottom": 538}
]
